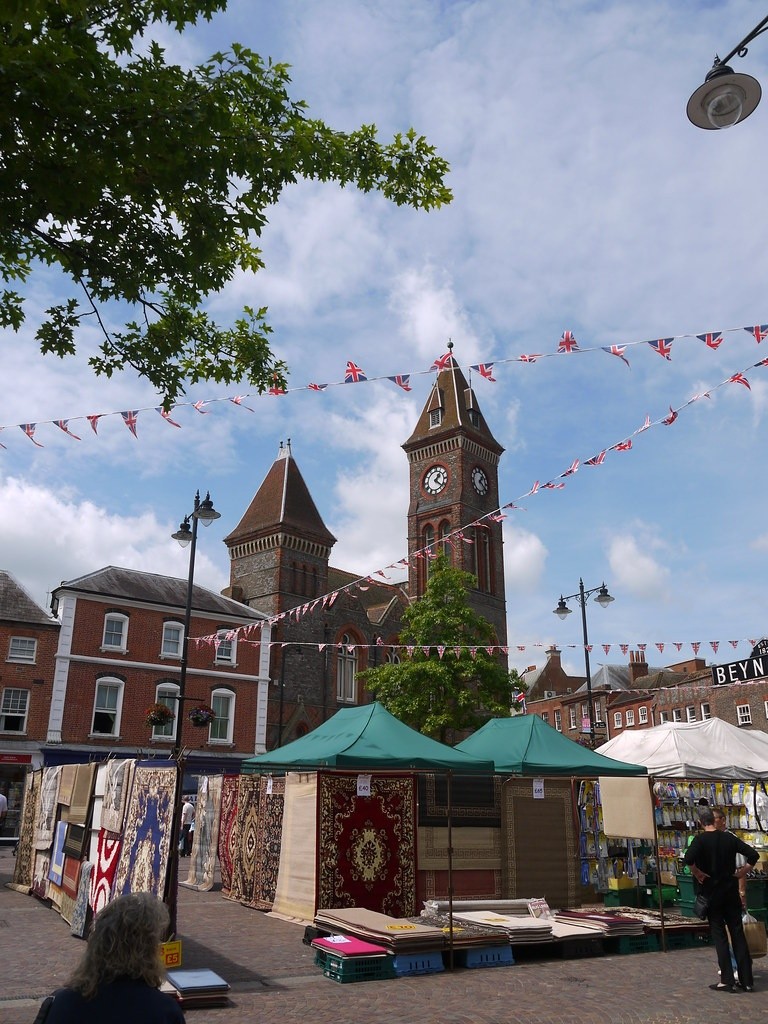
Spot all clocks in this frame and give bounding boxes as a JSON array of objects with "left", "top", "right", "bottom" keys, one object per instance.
[
  {"left": 422, "top": 465, "right": 448, "bottom": 495},
  {"left": 471, "top": 466, "right": 490, "bottom": 496}
]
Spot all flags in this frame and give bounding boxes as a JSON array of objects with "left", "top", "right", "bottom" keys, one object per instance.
[{"left": 513, "top": 691, "right": 527, "bottom": 710}]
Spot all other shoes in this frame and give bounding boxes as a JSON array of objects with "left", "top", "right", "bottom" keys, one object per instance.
[
  {"left": 733, "top": 970, "right": 738, "bottom": 981},
  {"left": 718, "top": 970, "right": 722, "bottom": 976},
  {"left": 709, "top": 982, "right": 737, "bottom": 993},
  {"left": 735, "top": 981, "right": 753, "bottom": 992}
]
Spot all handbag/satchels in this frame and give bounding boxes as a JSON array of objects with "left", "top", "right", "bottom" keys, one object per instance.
[
  {"left": 725, "top": 904, "right": 768, "bottom": 959},
  {"left": 693, "top": 894, "right": 709, "bottom": 921}
]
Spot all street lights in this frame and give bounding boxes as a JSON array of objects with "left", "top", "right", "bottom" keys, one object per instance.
[
  {"left": 552, "top": 577, "right": 615, "bottom": 747},
  {"left": 162, "top": 488, "right": 223, "bottom": 941}
]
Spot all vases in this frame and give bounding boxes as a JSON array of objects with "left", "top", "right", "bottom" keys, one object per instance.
[
  {"left": 149, "top": 718, "right": 165, "bottom": 726},
  {"left": 192, "top": 717, "right": 208, "bottom": 727}
]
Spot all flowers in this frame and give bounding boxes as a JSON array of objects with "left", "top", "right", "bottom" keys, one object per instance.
[
  {"left": 186, "top": 704, "right": 216, "bottom": 723},
  {"left": 143, "top": 703, "right": 176, "bottom": 728}
]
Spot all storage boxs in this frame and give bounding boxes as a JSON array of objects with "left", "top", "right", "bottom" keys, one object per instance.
[
  {"left": 560, "top": 930, "right": 714, "bottom": 956},
  {"left": 600, "top": 874, "right": 676, "bottom": 909},
  {"left": 676, "top": 876, "right": 768, "bottom": 930},
  {"left": 302, "top": 926, "right": 330, "bottom": 949}
]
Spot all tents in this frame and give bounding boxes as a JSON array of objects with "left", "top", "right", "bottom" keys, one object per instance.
[
  {"left": 454, "top": 714, "right": 648, "bottom": 777},
  {"left": 592, "top": 716, "right": 768, "bottom": 953},
  {"left": 244, "top": 702, "right": 496, "bottom": 971}
]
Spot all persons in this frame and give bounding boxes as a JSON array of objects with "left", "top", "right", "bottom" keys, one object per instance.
[
  {"left": 0, "top": 794, "right": 7, "bottom": 824},
  {"left": 179, "top": 795, "right": 197, "bottom": 858},
  {"left": 684, "top": 807, "right": 760, "bottom": 994},
  {"left": 33, "top": 891, "right": 186, "bottom": 1024}
]
